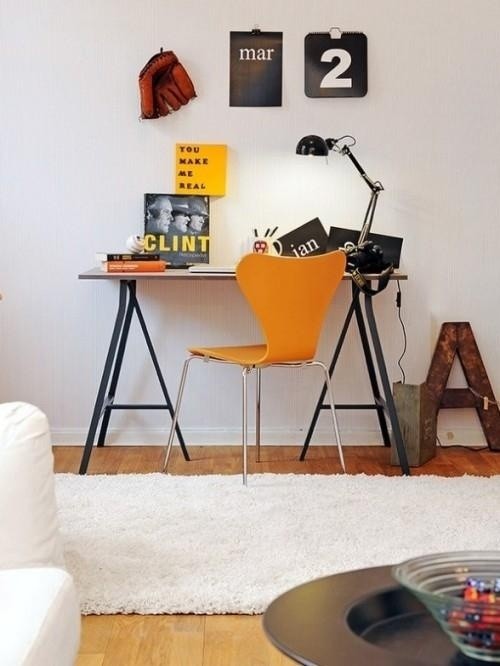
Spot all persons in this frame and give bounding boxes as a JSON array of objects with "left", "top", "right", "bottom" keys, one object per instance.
[{"left": 146, "top": 195, "right": 208, "bottom": 235}]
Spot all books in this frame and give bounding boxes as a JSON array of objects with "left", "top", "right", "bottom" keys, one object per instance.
[
  {"left": 143, "top": 193, "right": 209, "bottom": 264},
  {"left": 100, "top": 260, "right": 166, "bottom": 273},
  {"left": 175, "top": 141, "right": 229, "bottom": 197},
  {"left": 95, "top": 252, "right": 160, "bottom": 262}
]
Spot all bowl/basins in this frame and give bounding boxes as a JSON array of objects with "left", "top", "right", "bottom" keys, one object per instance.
[{"left": 391, "top": 549, "right": 500, "bottom": 664}]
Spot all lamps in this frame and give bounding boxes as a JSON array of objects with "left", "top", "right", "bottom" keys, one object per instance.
[{"left": 296, "top": 135, "right": 384, "bottom": 244}]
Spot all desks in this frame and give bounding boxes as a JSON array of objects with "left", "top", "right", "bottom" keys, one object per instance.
[
  {"left": 78, "top": 268, "right": 411, "bottom": 475},
  {"left": 262, "top": 565, "right": 500, "bottom": 666}
]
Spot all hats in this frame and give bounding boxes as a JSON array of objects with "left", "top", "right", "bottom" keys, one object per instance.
[{"left": 171, "top": 197, "right": 208, "bottom": 218}]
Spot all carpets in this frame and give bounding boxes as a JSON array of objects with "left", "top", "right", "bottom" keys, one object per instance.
[{"left": 54, "top": 473, "right": 500, "bottom": 615}]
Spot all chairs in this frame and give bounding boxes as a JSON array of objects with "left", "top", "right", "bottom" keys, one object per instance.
[
  {"left": 163, "top": 250, "right": 346, "bottom": 486},
  {"left": 0, "top": 401, "right": 82, "bottom": 666}
]
